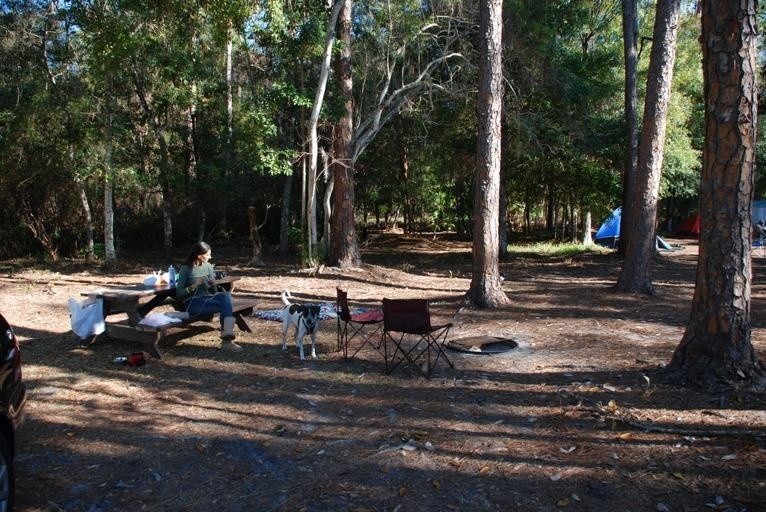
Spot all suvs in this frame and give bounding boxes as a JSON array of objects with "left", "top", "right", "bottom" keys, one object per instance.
[{"left": 2, "top": 307, "right": 29, "bottom": 512}]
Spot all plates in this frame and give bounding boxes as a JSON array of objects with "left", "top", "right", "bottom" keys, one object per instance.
[{"left": 317, "top": 302, "right": 333, "bottom": 313}]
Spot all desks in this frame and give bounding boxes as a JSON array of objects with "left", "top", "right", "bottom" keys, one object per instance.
[{"left": 80, "top": 276, "right": 239, "bottom": 359}]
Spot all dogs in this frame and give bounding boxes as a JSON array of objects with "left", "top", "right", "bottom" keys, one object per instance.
[{"left": 280, "top": 289, "right": 321, "bottom": 361}]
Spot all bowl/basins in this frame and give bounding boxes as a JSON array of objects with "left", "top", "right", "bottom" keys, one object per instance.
[{"left": 213, "top": 270, "right": 226, "bottom": 279}]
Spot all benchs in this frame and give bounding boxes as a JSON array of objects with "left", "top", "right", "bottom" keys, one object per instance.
[
  {"left": 70, "top": 296, "right": 175, "bottom": 348},
  {"left": 134, "top": 301, "right": 261, "bottom": 359}
]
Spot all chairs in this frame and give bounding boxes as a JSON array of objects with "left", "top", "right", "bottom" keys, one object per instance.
[
  {"left": 336, "top": 287, "right": 385, "bottom": 361},
  {"left": 382, "top": 297, "right": 452, "bottom": 380}
]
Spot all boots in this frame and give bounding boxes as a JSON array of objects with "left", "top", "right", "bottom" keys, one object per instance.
[
  {"left": 220, "top": 316, "right": 236, "bottom": 340},
  {"left": 221, "top": 340, "right": 243, "bottom": 354}
]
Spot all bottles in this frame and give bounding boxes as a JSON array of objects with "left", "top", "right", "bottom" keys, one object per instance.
[{"left": 168, "top": 264, "right": 176, "bottom": 288}]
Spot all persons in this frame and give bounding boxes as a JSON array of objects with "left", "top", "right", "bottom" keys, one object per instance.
[
  {"left": 752, "top": 218, "right": 766, "bottom": 239},
  {"left": 175, "top": 240, "right": 243, "bottom": 354}
]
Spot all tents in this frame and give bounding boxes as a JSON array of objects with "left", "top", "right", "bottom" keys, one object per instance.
[
  {"left": 594, "top": 205, "right": 623, "bottom": 250},
  {"left": 677, "top": 211, "right": 701, "bottom": 238},
  {"left": 662, "top": 216, "right": 683, "bottom": 234},
  {"left": 749, "top": 200, "right": 766, "bottom": 246}
]
